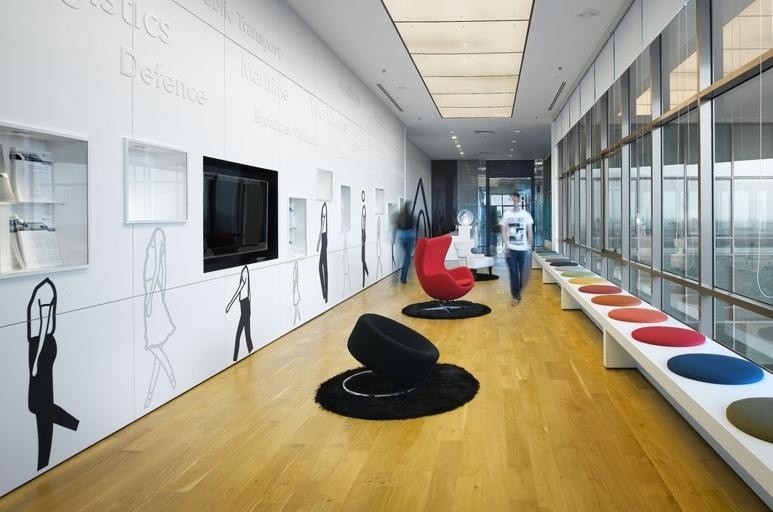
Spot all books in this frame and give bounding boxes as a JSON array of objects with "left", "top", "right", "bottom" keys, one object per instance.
[
  {"left": 8, "top": 151, "right": 54, "bottom": 201},
  {"left": 14, "top": 219, "right": 63, "bottom": 269},
  {"left": 8, "top": 220, "right": 26, "bottom": 270}
]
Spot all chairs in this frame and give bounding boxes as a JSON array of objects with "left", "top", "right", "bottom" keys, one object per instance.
[
  {"left": 414, "top": 235, "right": 475, "bottom": 314},
  {"left": 453, "top": 239, "right": 494, "bottom": 278}
]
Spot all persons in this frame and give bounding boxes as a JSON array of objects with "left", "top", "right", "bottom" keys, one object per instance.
[
  {"left": 497, "top": 192, "right": 534, "bottom": 307},
  {"left": 391, "top": 200, "right": 422, "bottom": 285}
]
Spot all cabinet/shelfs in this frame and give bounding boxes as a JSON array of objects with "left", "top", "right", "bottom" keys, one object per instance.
[{"left": 457, "top": 225, "right": 472, "bottom": 239}]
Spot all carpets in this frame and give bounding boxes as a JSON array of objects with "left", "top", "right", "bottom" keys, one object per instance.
[
  {"left": 401, "top": 300, "right": 491, "bottom": 319},
  {"left": 315, "top": 364, "right": 479, "bottom": 420},
  {"left": 472, "top": 273, "right": 498, "bottom": 280}
]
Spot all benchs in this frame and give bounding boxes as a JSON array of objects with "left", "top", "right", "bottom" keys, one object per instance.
[{"left": 531, "top": 249, "right": 773, "bottom": 512}]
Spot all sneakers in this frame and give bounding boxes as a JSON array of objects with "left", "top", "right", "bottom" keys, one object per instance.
[{"left": 511, "top": 297, "right": 519, "bottom": 306}]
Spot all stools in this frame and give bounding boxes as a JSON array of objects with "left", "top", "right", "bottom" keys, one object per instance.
[{"left": 341, "top": 313, "right": 440, "bottom": 396}]
